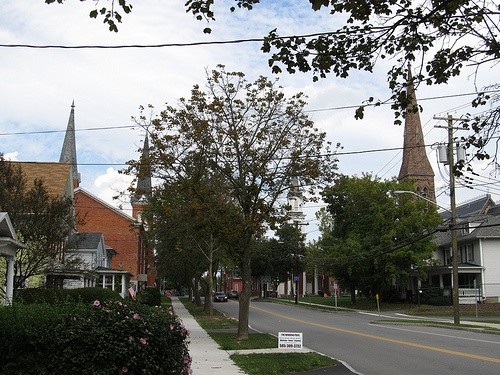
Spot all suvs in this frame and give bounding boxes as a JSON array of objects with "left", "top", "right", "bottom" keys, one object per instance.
[{"left": 228, "top": 290, "right": 238, "bottom": 299}]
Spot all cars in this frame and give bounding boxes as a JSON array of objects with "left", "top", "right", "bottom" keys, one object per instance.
[{"left": 214, "top": 291, "right": 228, "bottom": 302}]
